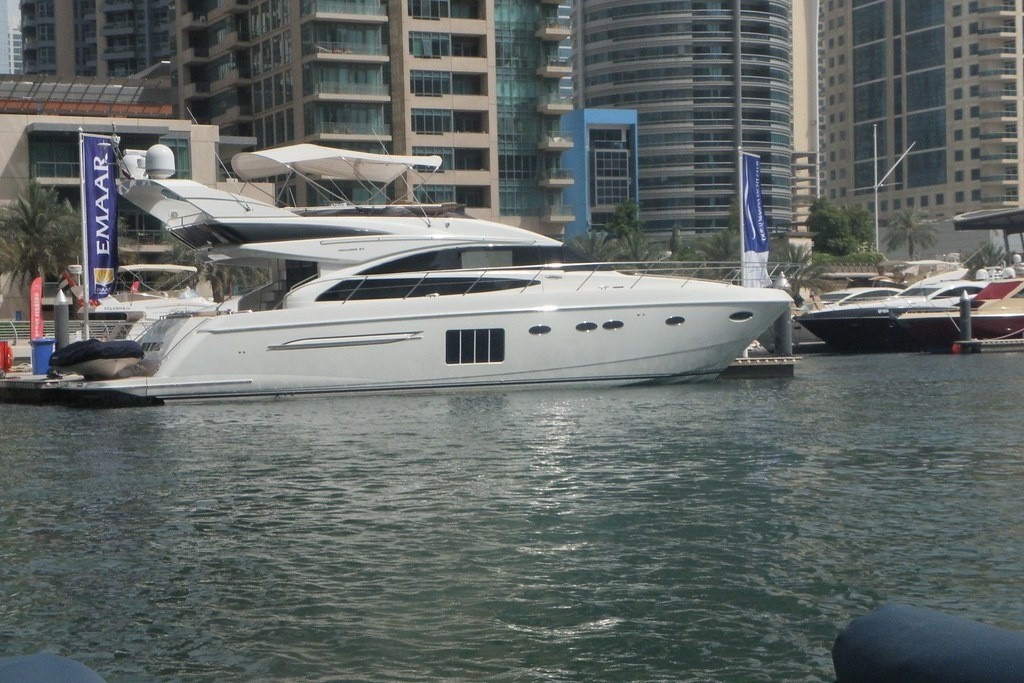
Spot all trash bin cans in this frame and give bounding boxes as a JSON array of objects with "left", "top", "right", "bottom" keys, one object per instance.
[{"left": 28, "top": 338, "right": 58, "bottom": 375}]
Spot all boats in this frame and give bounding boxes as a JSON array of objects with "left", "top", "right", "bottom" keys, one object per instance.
[
  {"left": 792, "top": 279, "right": 988, "bottom": 352},
  {"left": 0, "top": 106, "right": 805, "bottom": 406},
  {"left": 77, "top": 264, "right": 243, "bottom": 320},
  {"left": 755, "top": 287, "right": 904, "bottom": 354},
  {"left": 891, "top": 279, "right": 1024, "bottom": 353}
]
